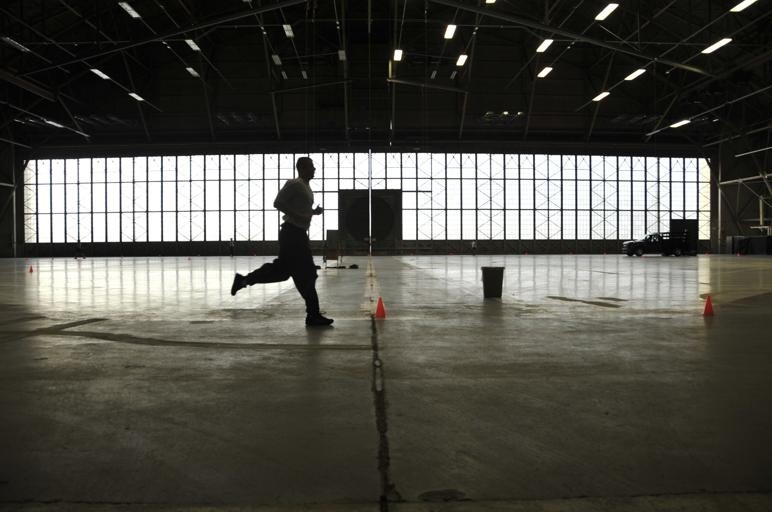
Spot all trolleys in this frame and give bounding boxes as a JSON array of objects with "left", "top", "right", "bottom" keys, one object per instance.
[{"left": 322, "top": 240, "right": 342, "bottom": 265}]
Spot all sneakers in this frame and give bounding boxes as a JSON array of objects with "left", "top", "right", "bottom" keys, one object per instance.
[
  {"left": 231, "top": 273, "right": 246, "bottom": 295},
  {"left": 306, "top": 313, "right": 333, "bottom": 326}
]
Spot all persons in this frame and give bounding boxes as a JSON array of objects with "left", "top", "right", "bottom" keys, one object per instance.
[
  {"left": 471, "top": 240, "right": 478, "bottom": 255},
  {"left": 230, "top": 156, "right": 336, "bottom": 326},
  {"left": 228, "top": 238, "right": 237, "bottom": 256},
  {"left": 74, "top": 240, "right": 86, "bottom": 259}
]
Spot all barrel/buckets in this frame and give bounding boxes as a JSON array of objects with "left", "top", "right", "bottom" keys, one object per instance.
[
  {"left": 661, "top": 239, "right": 673, "bottom": 257},
  {"left": 481, "top": 266, "right": 506, "bottom": 298}
]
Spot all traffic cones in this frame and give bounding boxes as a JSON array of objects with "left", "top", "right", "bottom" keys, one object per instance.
[
  {"left": 373, "top": 297, "right": 386, "bottom": 320},
  {"left": 701, "top": 295, "right": 716, "bottom": 316},
  {"left": 28, "top": 265, "right": 33, "bottom": 273}
]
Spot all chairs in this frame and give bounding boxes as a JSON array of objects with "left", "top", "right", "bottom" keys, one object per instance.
[{"left": 321, "top": 228, "right": 344, "bottom": 268}]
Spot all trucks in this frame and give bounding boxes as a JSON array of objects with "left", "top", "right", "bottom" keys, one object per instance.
[{"left": 623, "top": 232, "right": 688, "bottom": 257}]
